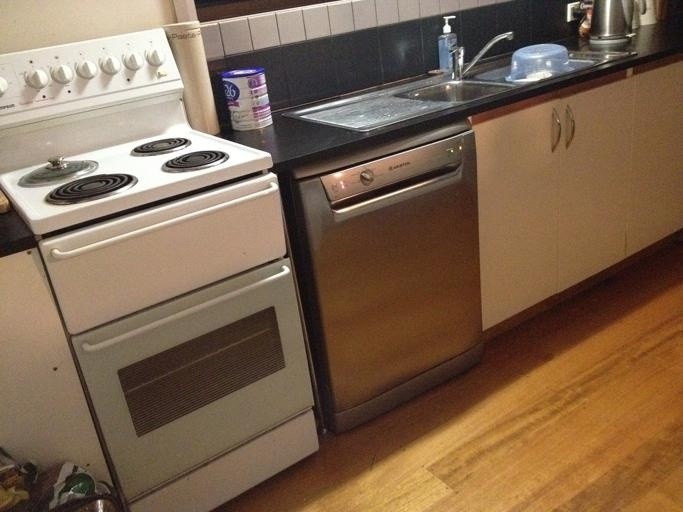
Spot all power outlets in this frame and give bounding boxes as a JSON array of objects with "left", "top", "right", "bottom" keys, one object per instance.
[{"left": 566, "top": 1, "right": 581, "bottom": 22}]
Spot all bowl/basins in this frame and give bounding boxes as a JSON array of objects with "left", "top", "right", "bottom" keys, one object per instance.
[{"left": 505, "top": 43, "right": 575, "bottom": 82}]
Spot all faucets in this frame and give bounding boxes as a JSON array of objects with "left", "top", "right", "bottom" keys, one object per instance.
[{"left": 449, "top": 30, "right": 515, "bottom": 82}]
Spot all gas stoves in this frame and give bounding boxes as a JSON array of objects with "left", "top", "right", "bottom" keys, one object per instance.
[{"left": 0, "top": 128, "right": 273, "bottom": 235}]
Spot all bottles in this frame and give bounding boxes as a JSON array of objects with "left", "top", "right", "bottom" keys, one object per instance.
[{"left": 162, "top": 20, "right": 221, "bottom": 135}]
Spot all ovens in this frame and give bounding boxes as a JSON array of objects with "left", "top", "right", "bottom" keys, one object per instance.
[{"left": 70, "top": 255, "right": 317, "bottom": 502}]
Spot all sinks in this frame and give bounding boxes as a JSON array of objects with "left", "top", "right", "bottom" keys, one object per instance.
[
  {"left": 470, "top": 57, "right": 600, "bottom": 85},
  {"left": 394, "top": 79, "right": 514, "bottom": 104}
]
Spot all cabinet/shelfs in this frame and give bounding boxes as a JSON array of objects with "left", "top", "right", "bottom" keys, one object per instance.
[
  {"left": 469, "top": 69, "right": 636, "bottom": 332},
  {"left": 626, "top": 60, "right": 683, "bottom": 259}
]
